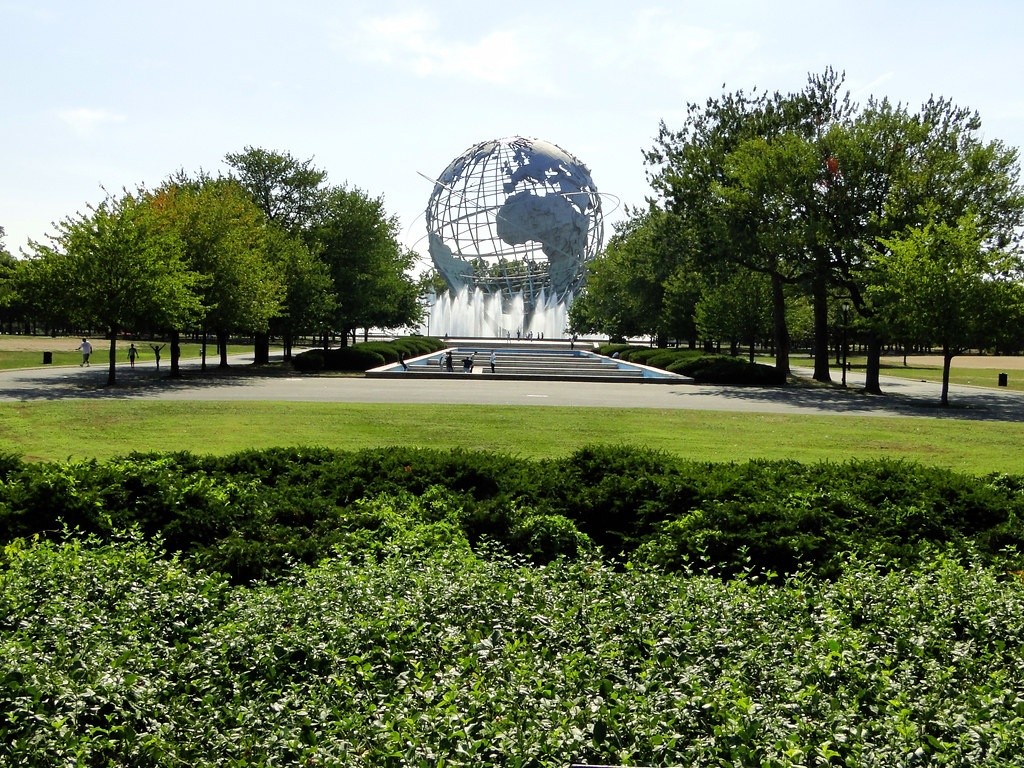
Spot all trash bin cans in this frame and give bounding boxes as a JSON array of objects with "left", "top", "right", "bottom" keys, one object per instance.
[
  {"left": 44, "top": 352, "right": 53, "bottom": 365},
  {"left": 998, "top": 373, "right": 1008, "bottom": 386}
]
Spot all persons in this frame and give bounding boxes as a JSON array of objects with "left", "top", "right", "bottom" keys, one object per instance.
[
  {"left": 397, "top": 352, "right": 407, "bottom": 371},
  {"left": 127, "top": 344, "right": 139, "bottom": 368},
  {"left": 150, "top": 344, "right": 166, "bottom": 369},
  {"left": 570, "top": 332, "right": 577, "bottom": 351},
  {"left": 506, "top": 330, "right": 544, "bottom": 343},
  {"left": 78, "top": 337, "right": 92, "bottom": 367},
  {"left": 490, "top": 349, "right": 496, "bottom": 373},
  {"left": 445, "top": 333, "right": 447, "bottom": 338},
  {"left": 461, "top": 351, "right": 478, "bottom": 373},
  {"left": 439, "top": 351, "right": 454, "bottom": 372}
]
